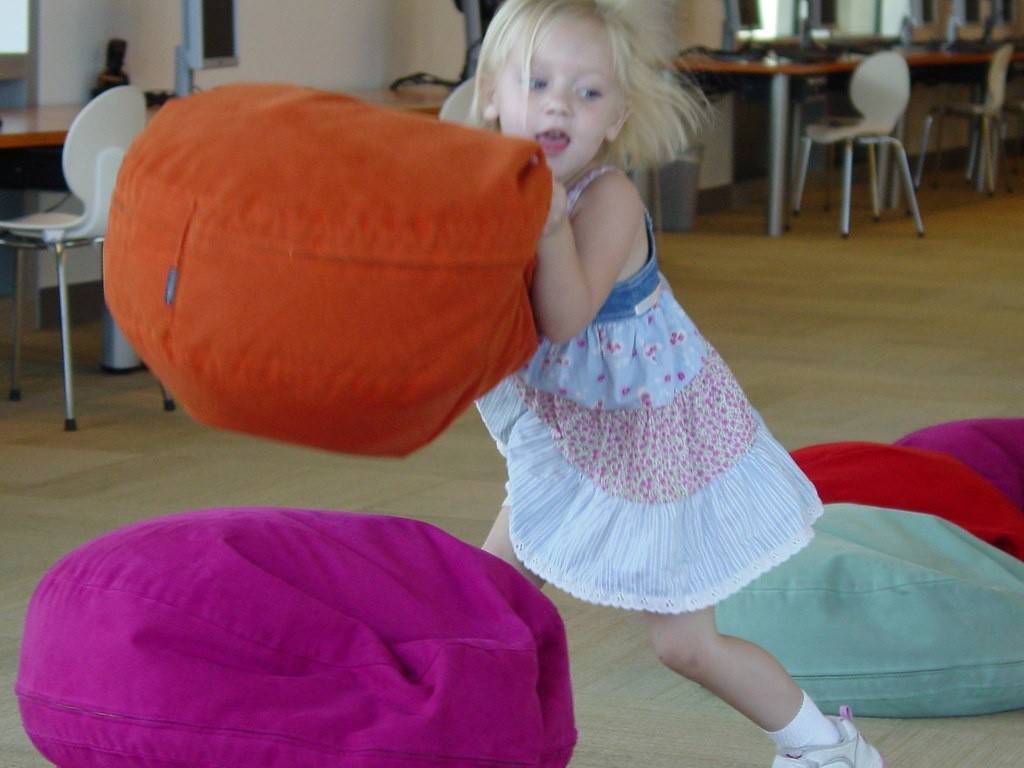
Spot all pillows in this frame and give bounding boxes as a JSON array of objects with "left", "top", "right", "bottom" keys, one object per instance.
[
  {"left": 714, "top": 504, "right": 1024, "bottom": 720},
  {"left": 17, "top": 504, "right": 584, "bottom": 768},
  {"left": 882, "top": 416, "right": 1024, "bottom": 515},
  {"left": 788, "top": 443, "right": 1024, "bottom": 560},
  {"left": 100, "top": 80, "right": 551, "bottom": 460}
]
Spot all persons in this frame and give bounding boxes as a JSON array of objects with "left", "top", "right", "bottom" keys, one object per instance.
[{"left": 428, "top": 0, "right": 887, "bottom": 768}]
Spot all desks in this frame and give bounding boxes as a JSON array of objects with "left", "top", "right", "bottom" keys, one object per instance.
[
  {"left": 0, "top": 86, "right": 456, "bottom": 374},
  {"left": 664, "top": 40, "right": 1024, "bottom": 241}
]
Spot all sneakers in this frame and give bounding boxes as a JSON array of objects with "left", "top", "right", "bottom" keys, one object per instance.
[{"left": 770, "top": 705, "right": 887, "bottom": 768}]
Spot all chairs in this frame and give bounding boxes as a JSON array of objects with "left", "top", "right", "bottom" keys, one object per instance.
[
  {"left": 0, "top": 85, "right": 184, "bottom": 437},
  {"left": 912, "top": 43, "right": 1015, "bottom": 194},
  {"left": 792, "top": 50, "right": 924, "bottom": 244},
  {"left": 965, "top": 98, "right": 1024, "bottom": 183}
]
